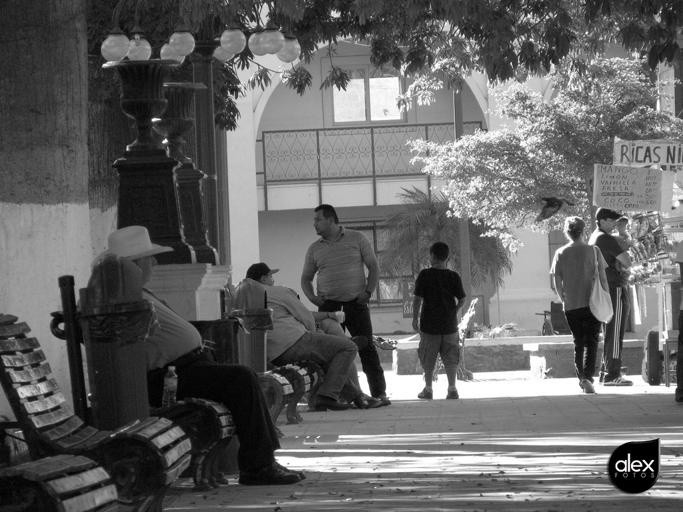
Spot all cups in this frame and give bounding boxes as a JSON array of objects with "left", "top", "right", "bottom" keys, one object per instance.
[{"left": 335, "top": 312, "right": 344, "bottom": 323}]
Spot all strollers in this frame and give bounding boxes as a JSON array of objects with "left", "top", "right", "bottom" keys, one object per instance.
[{"left": 421, "top": 297, "right": 479, "bottom": 381}]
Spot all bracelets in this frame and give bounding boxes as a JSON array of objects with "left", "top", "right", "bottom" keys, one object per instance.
[
  {"left": 327, "top": 312, "right": 330, "bottom": 318},
  {"left": 365, "top": 290, "right": 372, "bottom": 296}
]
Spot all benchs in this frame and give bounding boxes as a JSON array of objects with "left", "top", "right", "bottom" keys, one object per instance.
[{"left": 522, "top": 338, "right": 645, "bottom": 379}]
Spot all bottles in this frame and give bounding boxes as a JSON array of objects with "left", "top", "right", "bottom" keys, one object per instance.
[{"left": 162, "top": 366, "right": 178, "bottom": 408}]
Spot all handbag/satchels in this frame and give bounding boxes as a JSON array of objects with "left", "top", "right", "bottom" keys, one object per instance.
[{"left": 588, "top": 271, "right": 614, "bottom": 324}]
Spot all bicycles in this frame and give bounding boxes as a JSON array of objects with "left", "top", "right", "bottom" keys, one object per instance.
[{"left": 535, "top": 309, "right": 554, "bottom": 335}]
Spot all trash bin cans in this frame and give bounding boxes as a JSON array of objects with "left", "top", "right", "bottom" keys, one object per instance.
[
  {"left": 78, "top": 255, "right": 155, "bottom": 426},
  {"left": 188, "top": 320, "right": 239, "bottom": 366},
  {"left": 230, "top": 278, "right": 273, "bottom": 374}
]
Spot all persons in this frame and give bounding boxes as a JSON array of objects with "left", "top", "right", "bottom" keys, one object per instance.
[
  {"left": 550, "top": 213, "right": 610, "bottom": 393},
  {"left": 676, "top": 239, "right": 683, "bottom": 402},
  {"left": 611, "top": 217, "right": 631, "bottom": 274},
  {"left": 412, "top": 241, "right": 466, "bottom": 400},
  {"left": 283, "top": 287, "right": 382, "bottom": 409},
  {"left": 588, "top": 207, "right": 633, "bottom": 386},
  {"left": 246, "top": 262, "right": 358, "bottom": 412},
  {"left": 301, "top": 204, "right": 392, "bottom": 405},
  {"left": 90, "top": 225, "right": 306, "bottom": 485}
]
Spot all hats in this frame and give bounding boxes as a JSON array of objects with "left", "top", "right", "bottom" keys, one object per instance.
[
  {"left": 596, "top": 208, "right": 624, "bottom": 218},
  {"left": 246, "top": 262, "right": 280, "bottom": 280},
  {"left": 90, "top": 225, "right": 174, "bottom": 269}
]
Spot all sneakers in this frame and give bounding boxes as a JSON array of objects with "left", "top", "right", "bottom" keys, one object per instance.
[
  {"left": 418, "top": 389, "right": 433, "bottom": 399},
  {"left": 447, "top": 389, "right": 459, "bottom": 399},
  {"left": 578, "top": 375, "right": 634, "bottom": 392}
]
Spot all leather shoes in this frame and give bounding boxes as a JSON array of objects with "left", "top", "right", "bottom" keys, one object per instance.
[{"left": 239, "top": 394, "right": 391, "bottom": 486}]
